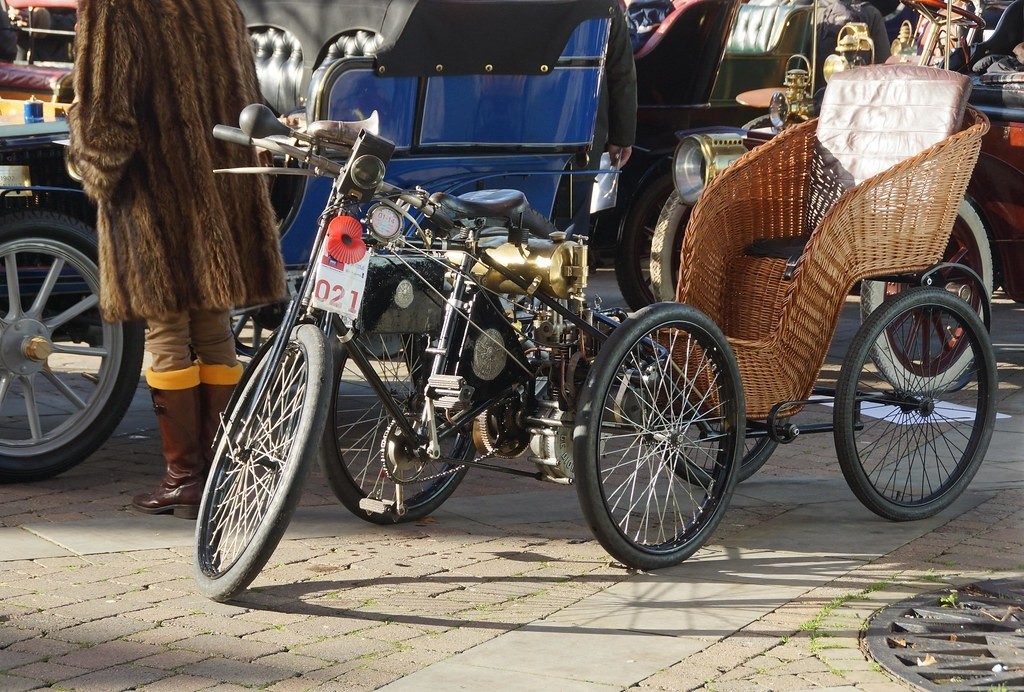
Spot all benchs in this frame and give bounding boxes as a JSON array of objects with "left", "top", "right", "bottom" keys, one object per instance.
[
  {"left": 0, "top": 60, "right": 75, "bottom": 94},
  {"left": 223, "top": 0, "right": 813, "bottom": 262}
]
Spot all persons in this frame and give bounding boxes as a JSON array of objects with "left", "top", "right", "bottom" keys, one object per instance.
[
  {"left": 67, "top": 0, "right": 288, "bottom": 519},
  {"left": 805, "top": 0, "right": 890, "bottom": 116},
  {"left": 552, "top": 0, "right": 637, "bottom": 245}
]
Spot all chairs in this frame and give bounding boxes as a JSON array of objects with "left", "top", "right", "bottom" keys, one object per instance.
[{"left": 654, "top": 61, "right": 992, "bottom": 425}]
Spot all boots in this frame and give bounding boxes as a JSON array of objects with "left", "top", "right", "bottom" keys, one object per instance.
[{"left": 131, "top": 360, "right": 245, "bottom": 520}]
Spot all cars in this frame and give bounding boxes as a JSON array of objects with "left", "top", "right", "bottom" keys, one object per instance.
[{"left": 0, "top": 1, "right": 1024, "bottom": 481}]
